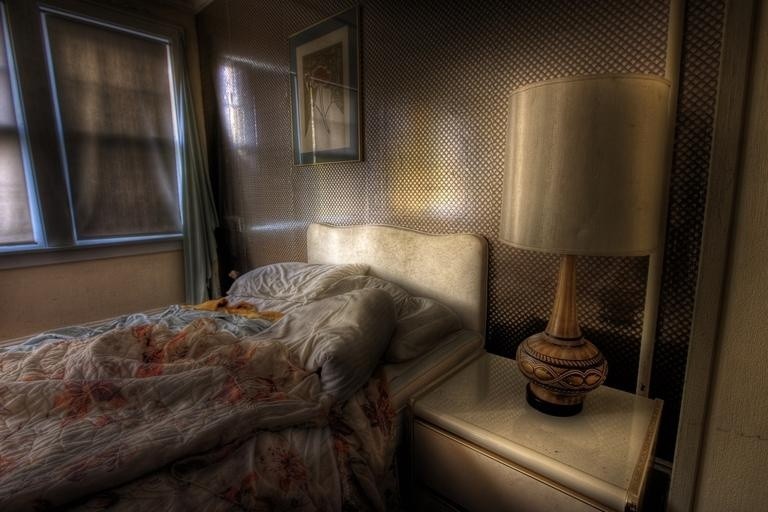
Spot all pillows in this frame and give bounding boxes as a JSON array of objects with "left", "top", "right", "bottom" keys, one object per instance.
[{"left": 227, "top": 258, "right": 464, "bottom": 365}]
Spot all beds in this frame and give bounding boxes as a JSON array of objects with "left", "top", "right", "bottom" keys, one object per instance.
[{"left": 0, "top": 221, "right": 492, "bottom": 512}]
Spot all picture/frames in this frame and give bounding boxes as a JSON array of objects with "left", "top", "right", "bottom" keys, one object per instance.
[{"left": 287, "top": 3, "right": 364, "bottom": 166}]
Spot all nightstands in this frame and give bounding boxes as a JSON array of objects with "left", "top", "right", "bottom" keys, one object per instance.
[{"left": 406, "top": 348, "right": 666, "bottom": 512}]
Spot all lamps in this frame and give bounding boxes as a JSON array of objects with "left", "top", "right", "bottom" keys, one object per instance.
[{"left": 496, "top": 71, "right": 676, "bottom": 418}]
